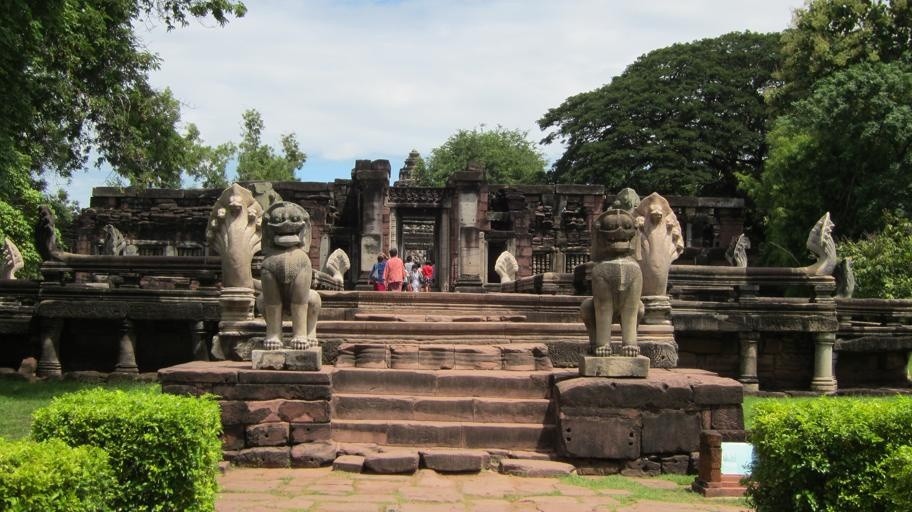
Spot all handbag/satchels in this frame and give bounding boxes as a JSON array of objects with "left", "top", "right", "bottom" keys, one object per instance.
[{"left": 372, "top": 268, "right": 379, "bottom": 280}]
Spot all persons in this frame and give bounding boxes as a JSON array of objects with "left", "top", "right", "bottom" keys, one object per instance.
[
  {"left": 402, "top": 255, "right": 436, "bottom": 292},
  {"left": 368, "top": 253, "right": 389, "bottom": 291},
  {"left": 382, "top": 248, "right": 406, "bottom": 292}
]
